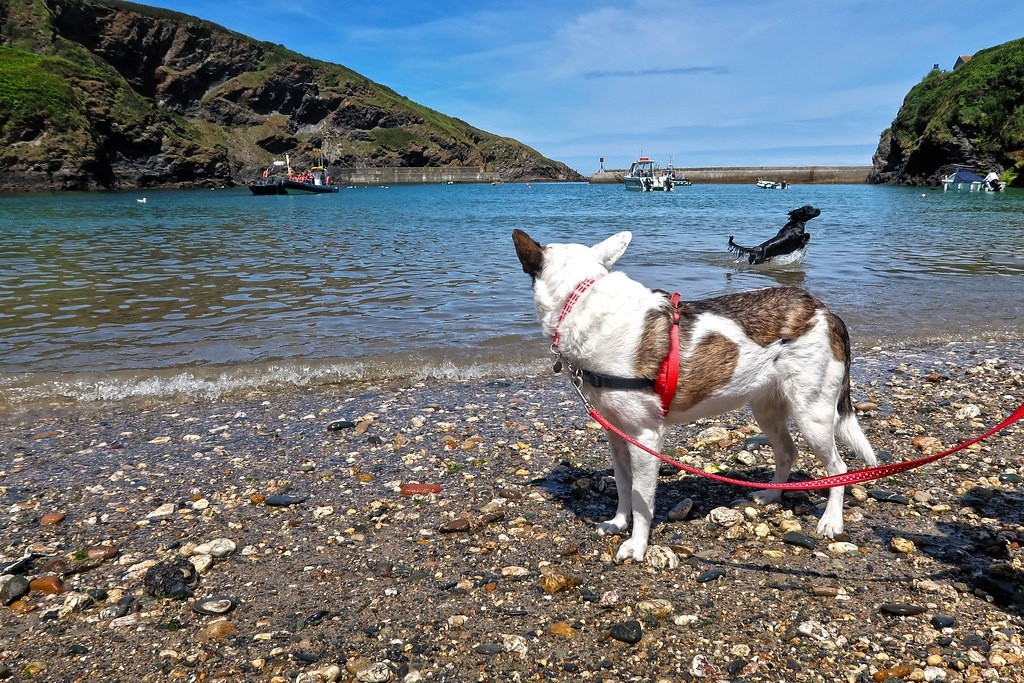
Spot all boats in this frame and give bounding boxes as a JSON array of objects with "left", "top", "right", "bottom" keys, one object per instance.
[
  {"left": 249, "top": 152, "right": 341, "bottom": 195},
  {"left": 672, "top": 177, "right": 693, "bottom": 186},
  {"left": 623, "top": 150, "right": 677, "bottom": 191},
  {"left": 939, "top": 166, "right": 1007, "bottom": 192},
  {"left": 756, "top": 176, "right": 791, "bottom": 190}
]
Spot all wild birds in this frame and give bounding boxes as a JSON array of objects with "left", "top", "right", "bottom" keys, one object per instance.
[{"left": 137, "top": 198, "right": 148, "bottom": 203}]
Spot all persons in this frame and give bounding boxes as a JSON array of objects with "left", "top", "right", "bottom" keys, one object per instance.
[
  {"left": 326, "top": 174, "right": 332, "bottom": 186},
  {"left": 291, "top": 171, "right": 314, "bottom": 183}
]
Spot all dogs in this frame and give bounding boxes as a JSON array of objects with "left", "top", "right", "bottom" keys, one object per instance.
[
  {"left": 512, "top": 228, "right": 879, "bottom": 564},
  {"left": 726, "top": 205, "right": 822, "bottom": 266}
]
